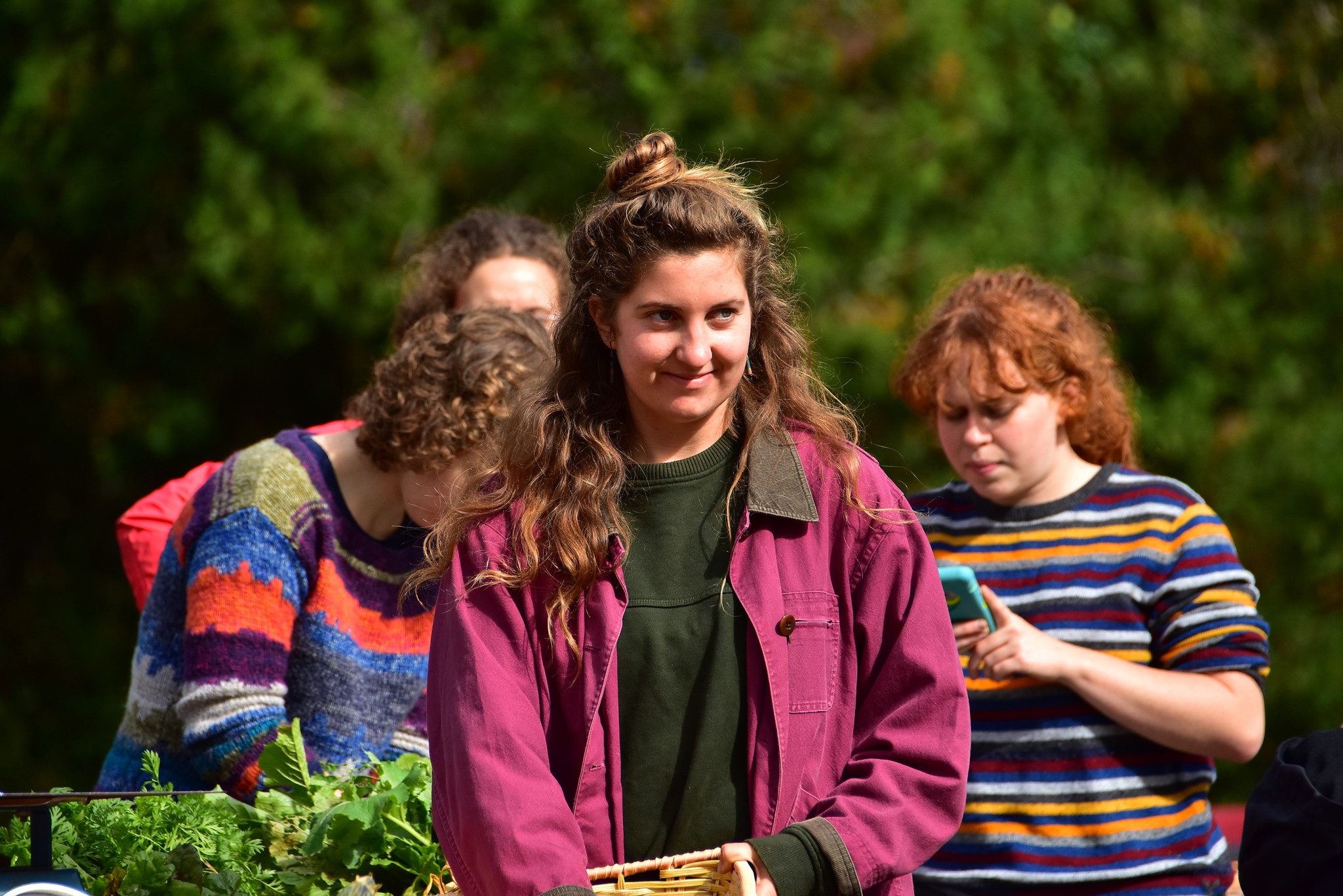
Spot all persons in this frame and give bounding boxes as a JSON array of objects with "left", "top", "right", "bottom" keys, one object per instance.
[
  {"left": 886, "top": 265, "right": 1271, "bottom": 896},
  {"left": 114, "top": 204, "right": 574, "bottom": 618},
  {"left": 416, "top": 130, "right": 971, "bottom": 896},
  {"left": 92, "top": 303, "right": 555, "bottom": 807}
]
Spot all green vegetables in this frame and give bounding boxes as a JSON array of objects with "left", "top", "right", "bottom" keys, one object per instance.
[{"left": 0, "top": 716, "right": 461, "bottom": 896}]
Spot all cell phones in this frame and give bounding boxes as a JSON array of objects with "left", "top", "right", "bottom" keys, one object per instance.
[{"left": 938, "top": 565, "right": 995, "bottom": 639}]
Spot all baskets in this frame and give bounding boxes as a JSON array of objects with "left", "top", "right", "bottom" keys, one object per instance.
[{"left": 431, "top": 844, "right": 759, "bottom": 896}]
